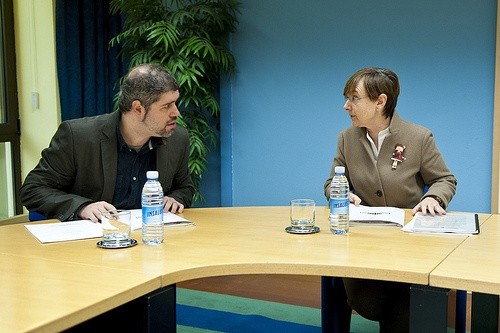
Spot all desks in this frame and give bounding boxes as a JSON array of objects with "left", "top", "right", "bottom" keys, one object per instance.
[{"left": 0, "top": 204, "right": 500, "bottom": 333}]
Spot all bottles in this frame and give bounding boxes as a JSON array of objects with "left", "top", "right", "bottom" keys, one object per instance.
[
  {"left": 141, "top": 171, "right": 164, "bottom": 245},
  {"left": 329, "top": 166, "right": 350, "bottom": 234}
]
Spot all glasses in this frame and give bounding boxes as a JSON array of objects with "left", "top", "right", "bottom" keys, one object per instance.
[{"left": 344, "top": 94, "right": 371, "bottom": 105}]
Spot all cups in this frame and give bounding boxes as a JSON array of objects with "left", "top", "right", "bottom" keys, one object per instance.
[
  {"left": 101, "top": 209, "right": 131, "bottom": 245},
  {"left": 290, "top": 199, "right": 316, "bottom": 230}
]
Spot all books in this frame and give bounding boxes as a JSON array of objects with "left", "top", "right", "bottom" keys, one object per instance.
[
  {"left": 349, "top": 203, "right": 404, "bottom": 227},
  {"left": 115, "top": 206, "right": 192, "bottom": 230},
  {"left": 402, "top": 212, "right": 481, "bottom": 235}
]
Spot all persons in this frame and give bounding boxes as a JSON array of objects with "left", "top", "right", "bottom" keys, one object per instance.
[
  {"left": 324, "top": 68, "right": 458, "bottom": 217},
  {"left": 20, "top": 63, "right": 195, "bottom": 223}
]
